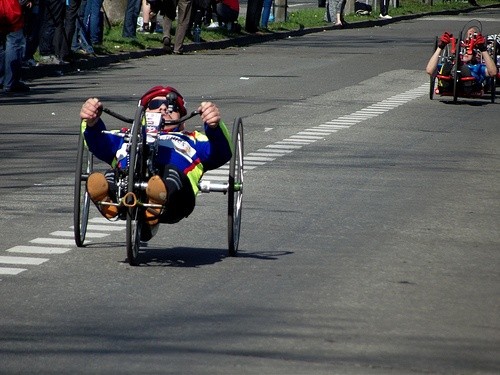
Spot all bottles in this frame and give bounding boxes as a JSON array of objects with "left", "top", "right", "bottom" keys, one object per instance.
[{"left": 194, "top": 25, "right": 201, "bottom": 44}]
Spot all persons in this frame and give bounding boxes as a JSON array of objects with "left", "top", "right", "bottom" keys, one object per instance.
[
  {"left": 0, "top": 0, "right": 400, "bottom": 98},
  {"left": 426, "top": 27, "right": 498, "bottom": 98},
  {"left": 78, "top": 85, "right": 233, "bottom": 225}
]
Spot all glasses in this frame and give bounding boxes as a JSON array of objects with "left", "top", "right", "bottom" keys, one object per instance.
[{"left": 147, "top": 99, "right": 180, "bottom": 113}]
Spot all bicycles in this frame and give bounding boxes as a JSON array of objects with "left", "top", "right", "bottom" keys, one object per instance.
[
  {"left": 74, "top": 105, "right": 246, "bottom": 264},
  {"left": 429, "top": 19, "right": 500, "bottom": 103}
]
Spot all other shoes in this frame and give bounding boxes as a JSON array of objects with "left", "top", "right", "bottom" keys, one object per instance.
[
  {"left": 260, "top": 28, "right": 274, "bottom": 33},
  {"left": 443, "top": 62, "right": 472, "bottom": 93}
]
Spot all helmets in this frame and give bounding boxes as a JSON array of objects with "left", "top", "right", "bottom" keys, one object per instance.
[
  {"left": 486, "top": 34, "right": 500, "bottom": 45},
  {"left": 138, "top": 86, "right": 188, "bottom": 119},
  {"left": 460, "top": 40, "right": 471, "bottom": 47}
]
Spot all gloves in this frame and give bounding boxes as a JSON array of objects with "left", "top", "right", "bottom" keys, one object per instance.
[
  {"left": 438, "top": 31, "right": 453, "bottom": 50},
  {"left": 474, "top": 32, "right": 488, "bottom": 52}
]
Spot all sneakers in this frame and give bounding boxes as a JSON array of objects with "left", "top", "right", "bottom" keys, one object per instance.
[
  {"left": 86, "top": 172, "right": 120, "bottom": 222},
  {"left": 146, "top": 175, "right": 167, "bottom": 225},
  {"left": 172, "top": 50, "right": 183, "bottom": 55},
  {"left": 163, "top": 38, "right": 170, "bottom": 50},
  {"left": 40, "top": 55, "right": 60, "bottom": 64},
  {"left": 3, "top": 82, "right": 30, "bottom": 96},
  {"left": 380, "top": 14, "right": 392, "bottom": 19}
]
